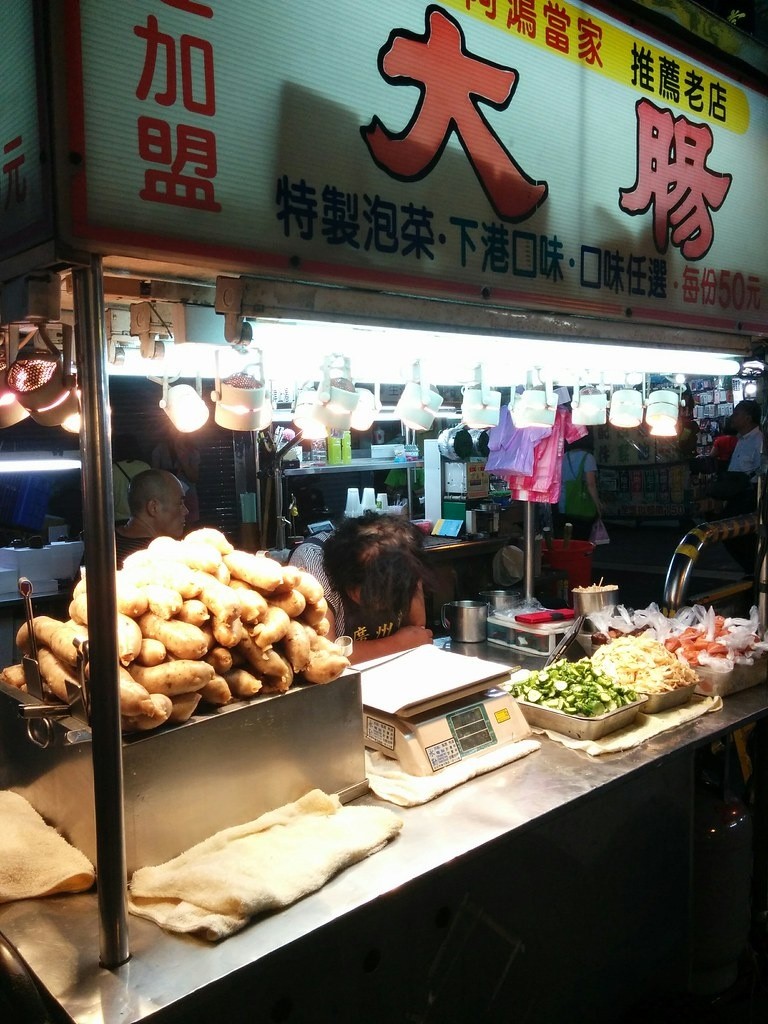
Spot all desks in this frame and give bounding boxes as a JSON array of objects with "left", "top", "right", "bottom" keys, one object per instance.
[{"left": 422, "top": 534, "right": 511, "bottom": 619}]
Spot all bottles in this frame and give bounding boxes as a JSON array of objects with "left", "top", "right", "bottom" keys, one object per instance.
[
  {"left": 312, "top": 438, "right": 327, "bottom": 465},
  {"left": 327, "top": 436, "right": 341, "bottom": 465},
  {"left": 342, "top": 431, "right": 352, "bottom": 464}
]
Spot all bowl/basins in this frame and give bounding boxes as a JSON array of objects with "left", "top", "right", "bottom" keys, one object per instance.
[{"left": 566, "top": 626, "right": 592, "bottom": 657}]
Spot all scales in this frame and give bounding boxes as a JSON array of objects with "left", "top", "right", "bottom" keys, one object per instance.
[{"left": 347, "top": 643, "right": 532, "bottom": 778}]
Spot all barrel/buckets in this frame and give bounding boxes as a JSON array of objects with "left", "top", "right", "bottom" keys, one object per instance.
[
  {"left": 515, "top": 567, "right": 568, "bottom": 608},
  {"left": 540, "top": 539, "right": 596, "bottom": 588}
]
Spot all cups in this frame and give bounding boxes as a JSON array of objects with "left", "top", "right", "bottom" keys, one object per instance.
[
  {"left": 345, "top": 487, "right": 403, "bottom": 520},
  {"left": 479, "top": 590, "right": 521, "bottom": 609},
  {"left": 571, "top": 589, "right": 618, "bottom": 620},
  {"left": 441, "top": 600, "right": 488, "bottom": 643}
]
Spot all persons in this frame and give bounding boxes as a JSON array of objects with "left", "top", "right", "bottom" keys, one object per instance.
[
  {"left": 113, "top": 428, "right": 200, "bottom": 573},
  {"left": 289, "top": 510, "right": 435, "bottom": 666},
  {"left": 384, "top": 431, "right": 435, "bottom": 512},
  {"left": 558, "top": 436, "right": 602, "bottom": 541},
  {"left": 705, "top": 400, "right": 768, "bottom": 572}
]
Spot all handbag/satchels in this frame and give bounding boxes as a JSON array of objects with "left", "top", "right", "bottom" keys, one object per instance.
[
  {"left": 709, "top": 470, "right": 749, "bottom": 502},
  {"left": 589, "top": 517, "right": 610, "bottom": 545},
  {"left": 565, "top": 452, "right": 601, "bottom": 520}
]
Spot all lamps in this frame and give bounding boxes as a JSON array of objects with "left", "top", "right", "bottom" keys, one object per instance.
[
  {"left": 0, "top": 322, "right": 82, "bottom": 434},
  {"left": 158, "top": 375, "right": 209, "bottom": 433},
  {"left": 507, "top": 366, "right": 558, "bottom": 429},
  {"left": 571, "top": 369, "right": 686, "bottom": 436},
  {"left": 393, "top": 359, "right": 443, "bottom": 431},
  {"left": 291, "top": 349, "right": 382, "bottom": 440},
  {"left": 210, "top": 345, "right": 278, "bottom": 432},
  {"left": 459, "top": 364, "right": 502, "bottom": 428}
]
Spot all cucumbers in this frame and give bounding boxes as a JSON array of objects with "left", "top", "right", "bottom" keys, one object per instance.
[{"left": 504, "top": 656, "right": 638, "bottom": 718}]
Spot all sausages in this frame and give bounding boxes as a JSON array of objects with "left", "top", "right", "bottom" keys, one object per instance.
[{"left": 0, "top": 526, "right": 351, "bottom": 732}]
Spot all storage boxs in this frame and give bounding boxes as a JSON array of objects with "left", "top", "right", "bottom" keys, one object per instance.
[
  {"left": 0, "top": 541, "right": 84, "bottom": 595},
  {"left": 689, "top": 654, "right": 767, "bottom": 698}
]
